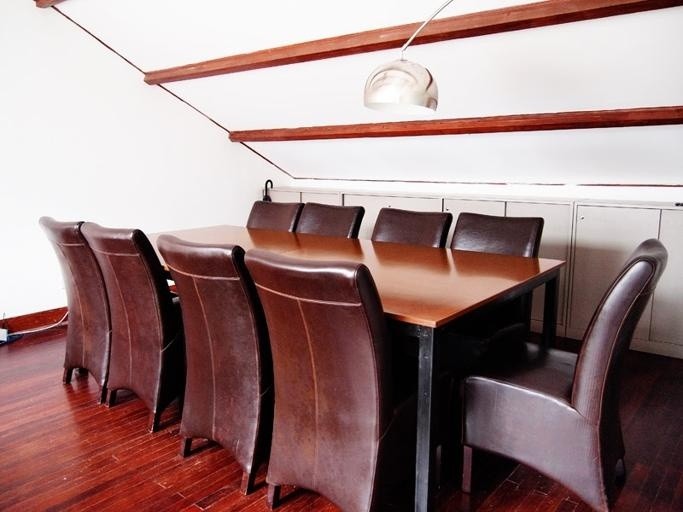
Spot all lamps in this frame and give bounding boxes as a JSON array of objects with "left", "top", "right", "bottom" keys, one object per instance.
[{"left": 359, "top": 0, "right": 455, "bottom": 118}]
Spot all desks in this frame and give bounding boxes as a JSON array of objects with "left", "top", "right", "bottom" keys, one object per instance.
[{"left": 144, "top": 224, "right": 565, "bottom": 511}]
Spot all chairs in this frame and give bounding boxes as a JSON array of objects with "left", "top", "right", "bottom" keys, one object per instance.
[
  {"left": 369, "top": 206, "right": 454, "bottom": 250},
  {"left": 293, "top": 202, "right": 365, "bottom": 240},
  {"left": 77, "top": 219, "right": 183, "bottom": 434},
  {"left": 455, "top": 237, "right": 667, "bottom": 510},
  {"left": 37, "top": 214, "right": 114, "bottom": 405},
  {"left": 154, "top": 229, "right": 274, "bottom": 497},
  {"left": 449, "top": 210, "right": 546, "bottom": 368},
  {"left": 246, "top": 201, "right": 306, "bottom": 232},
  {"left": 243, "top": 245, "right": 414, "bottom": 512}
]
